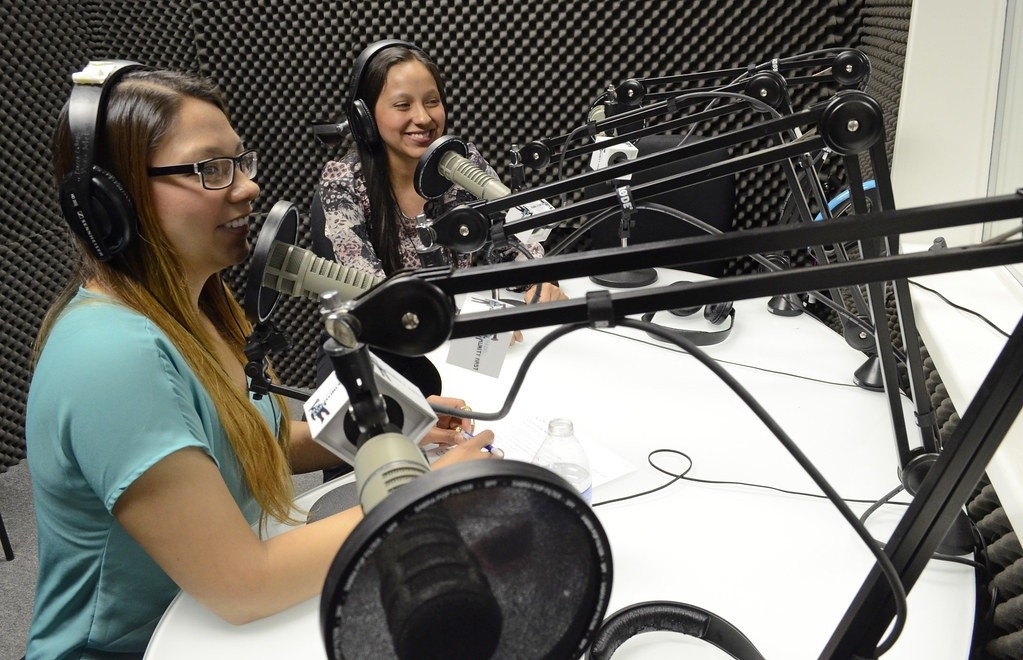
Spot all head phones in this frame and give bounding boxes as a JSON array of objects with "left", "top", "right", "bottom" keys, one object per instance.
[
  {"left": 55, "top": 57, "right": 158, "bottom": 263},
  {"left": 346, "top": 39, "right": 426, "bottom": 147},
  {"left": 641, "top": 281, "right": 736, "bottom": 346}
]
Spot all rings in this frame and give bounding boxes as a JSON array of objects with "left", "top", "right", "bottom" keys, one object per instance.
[
  {"left": 459, "top": 405, "right": 476, "bottom": 427},
  {"left": 452, "top": 427, "right": 464, "bottom": 432}
]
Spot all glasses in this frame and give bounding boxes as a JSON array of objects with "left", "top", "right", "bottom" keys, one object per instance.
[{"left": 146, "top": 149, "right": 260, "bottom": 191}]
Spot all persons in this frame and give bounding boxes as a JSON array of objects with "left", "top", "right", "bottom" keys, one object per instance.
[
  {"left": 318, "top": 38, "right": 573, "bottom": 348},
  {"left": 21, "top": 68, "right": 504, "bottom": 660}
]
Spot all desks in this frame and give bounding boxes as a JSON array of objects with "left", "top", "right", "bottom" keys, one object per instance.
[{"left": 143, "top": 263, "right": 981, "bottom": 660}]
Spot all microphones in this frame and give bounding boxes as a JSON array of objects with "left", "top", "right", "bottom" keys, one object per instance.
[
  {"left": 262, "top": 241, "right": 386, "bottom": 304},
  {"left": 353, "top": 431, "right": 502, "bottom": 660},
  {"left": 437, "top": 150, "right": 511, "bottom": 202}
]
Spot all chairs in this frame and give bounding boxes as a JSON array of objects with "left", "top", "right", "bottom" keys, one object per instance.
[
  {"left": 563, "top": 135, "right": 736, "bottom": 280},
  {"left": 309, "top": 186, "right": 337, "bottom": 265}
]
